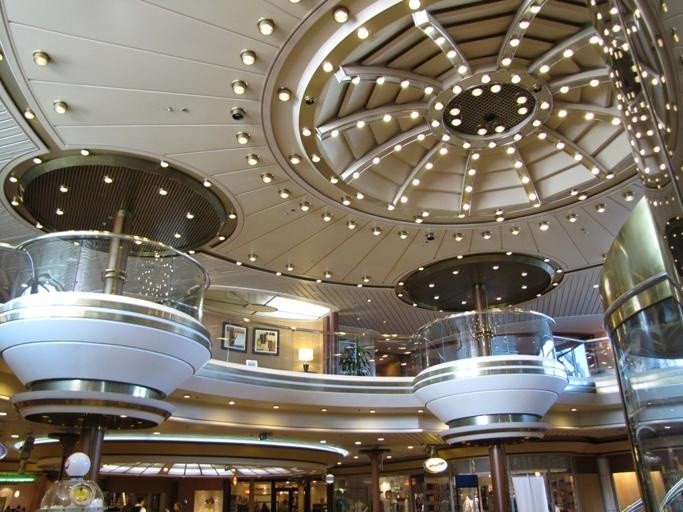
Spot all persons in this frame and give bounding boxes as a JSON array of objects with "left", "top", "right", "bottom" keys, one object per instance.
[{"left": 130, "top": 498, "right": 147, "bottom": 512}]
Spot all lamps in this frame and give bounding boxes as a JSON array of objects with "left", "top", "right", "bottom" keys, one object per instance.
[
  {"left": 298, "top": 347, "right": 314, "bottom": 372},
  {"left": 229, "top": 1, "right": 683, "bottom": 313},
  {"left": 6, "top": 51, "right": 237, "bottom": 260}
]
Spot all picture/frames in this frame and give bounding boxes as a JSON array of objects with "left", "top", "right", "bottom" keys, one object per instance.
[
  {"left": 252, "top": 327, "right": 279, "bottom": 356},
  {"left": 221, "top": 321, "right": 248, "bottom": 353}
]
